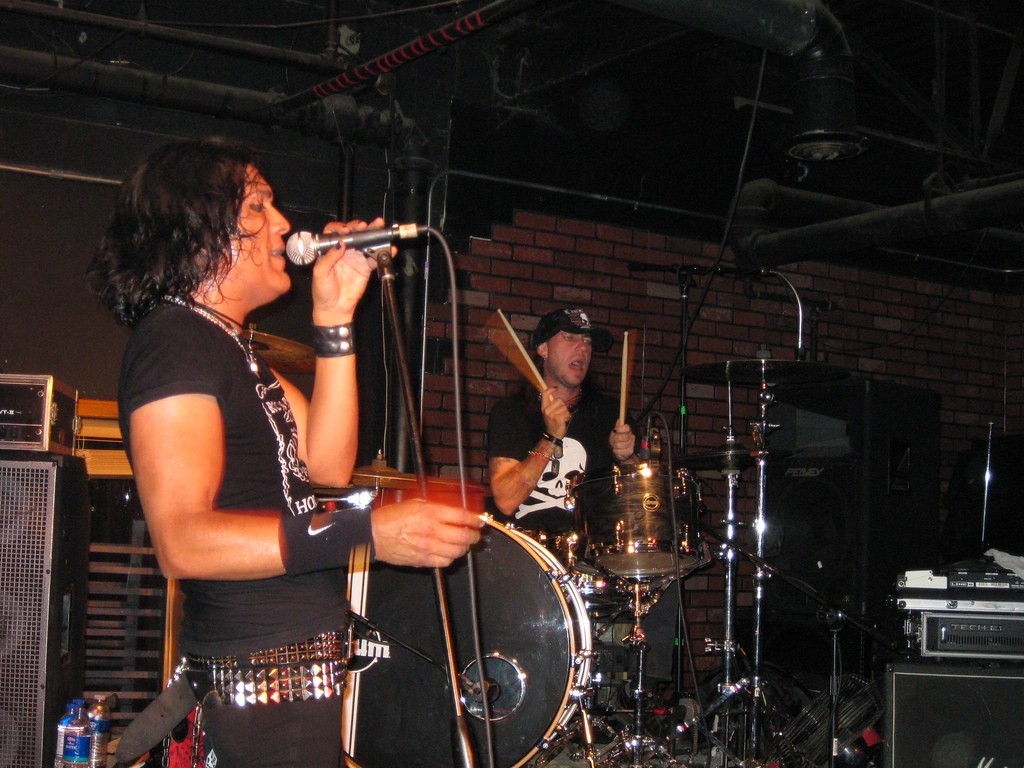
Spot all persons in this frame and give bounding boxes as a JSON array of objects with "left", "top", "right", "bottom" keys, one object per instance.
[
  {"left": 85, "top": 136, "right": 486, "bottom": 768},
  {"left": 483, "top": 307, "right": 683, "bottom": 708}
]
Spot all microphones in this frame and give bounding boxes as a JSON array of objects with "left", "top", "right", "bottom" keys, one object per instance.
[
  {"left": 286, "top": 220, "right": 428, "bottom": 264},
  {"left": 472, "top": 679, "right": 500, "bottom": 703}
]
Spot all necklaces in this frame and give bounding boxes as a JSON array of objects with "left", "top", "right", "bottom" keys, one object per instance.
[
  {"left": 163, "top": 294, "right": 259, "bottom": 372},
  {"left": 538, "top": 386, "right": 582, "bottom": 408}
]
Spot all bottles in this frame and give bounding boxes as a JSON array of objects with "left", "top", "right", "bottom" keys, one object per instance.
[{"left": 55, "top": 693, "right": 110, "bottom": 768}]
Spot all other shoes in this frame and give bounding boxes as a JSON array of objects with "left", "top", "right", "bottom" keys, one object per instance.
[{"left": 615, "top": 683, "right": 671, "bottom": 739}]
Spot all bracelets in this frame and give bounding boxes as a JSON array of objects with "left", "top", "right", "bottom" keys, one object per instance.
[
  {"left": 542, "top": 432, "right": 563, "bottom": 447},
  {"left": 311, "top": 319, "right": 356, "bottom": 357},
  {"left": 528, "top": 451, "right": 554, "bottom": 461},
  {"left": 282, "top": 505, "right": 376, "bottom": 576}
]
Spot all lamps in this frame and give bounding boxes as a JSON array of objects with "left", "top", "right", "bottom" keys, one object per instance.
[{"left": 783, "top": 52, "right": 871, "bottom": 163}]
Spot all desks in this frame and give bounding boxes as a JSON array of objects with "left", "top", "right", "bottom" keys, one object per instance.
[{"left": 353, "top": 470, "right": 486, "bottom": 514}]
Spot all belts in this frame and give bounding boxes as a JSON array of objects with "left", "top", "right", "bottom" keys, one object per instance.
[{"left": 115, "top": 626, "right": 349, "bottom": 761}]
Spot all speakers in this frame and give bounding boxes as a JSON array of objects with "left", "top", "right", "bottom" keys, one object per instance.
[
  {"left": 763, "top": 372, "right": 941, "bottom": 690},
  {"left": 884, "top": 663, "right": 1024, "bottom": 768},
  {"left": 0, "top": 449, "right": 92, "bottom": 768}
]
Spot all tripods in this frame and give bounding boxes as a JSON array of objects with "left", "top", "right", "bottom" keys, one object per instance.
[{"left": 537, "top": 371, "right": 795, "bottom": 768}]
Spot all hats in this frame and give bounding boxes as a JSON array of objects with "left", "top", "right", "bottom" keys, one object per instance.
[{"left": 533, "top": 307, "right": 613, "bottom": 355}]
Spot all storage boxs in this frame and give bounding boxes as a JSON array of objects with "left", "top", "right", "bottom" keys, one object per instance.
[{"left": 0, "top": 373, "right": 81, "bottom": 456}]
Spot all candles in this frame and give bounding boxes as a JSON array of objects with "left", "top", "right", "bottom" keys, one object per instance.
[{"left": 376, "top": 448, "right": 383, "bottom": 460}]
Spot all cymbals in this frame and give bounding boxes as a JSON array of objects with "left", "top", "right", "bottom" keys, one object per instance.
[
  {"left": 352, "top": 451, "right": 483, "bottom": 489},
  {"left": 240, "top": 329, "right": 315, "bottom": 373},
  {"left": 681, "top": 359, "right": 849, "bottom": 385}
]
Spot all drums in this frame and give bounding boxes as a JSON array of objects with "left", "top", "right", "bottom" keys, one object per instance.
[
  {"left": 338, "top": 498, "right": 591, "bottom": 768},
  {"left": 566, "top": 461, "right": 708, "bottom": 575}
]
